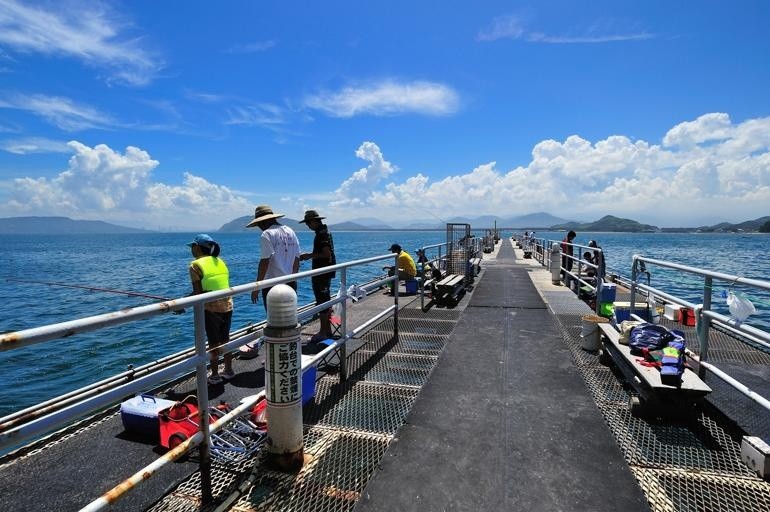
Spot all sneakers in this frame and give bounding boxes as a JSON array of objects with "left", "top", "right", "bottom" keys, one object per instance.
[
  {"left": 207, "top": 367, "right": 235, "bottom": 385},
  {"left": 307, "top": 329, "right": 333, "bottom": 343}
]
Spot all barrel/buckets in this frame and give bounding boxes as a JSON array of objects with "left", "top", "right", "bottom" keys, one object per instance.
[
  {"left": 579, "top": 314, "right": 610, "bottom": 352},
  {"left": 600, "top": 282, "right": 617, "bottom": 303}
]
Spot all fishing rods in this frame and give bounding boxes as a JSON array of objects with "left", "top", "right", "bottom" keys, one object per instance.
[
  {"left": 423, "top": 210, "right": 464, "bottom": 238},
  {"left": 4, "top": 277, "right": 186, "bottom": 315}
]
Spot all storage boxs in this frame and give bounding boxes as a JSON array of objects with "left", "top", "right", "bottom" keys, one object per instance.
[
  {"left": 121, "top": 395, "right": 177, "bottom": 439},
  {"left": 613, "top": 302, "right": 648, "bottom": 323},
  {"left": 301, "top": 354, "right": 318, "bottom": 404}
]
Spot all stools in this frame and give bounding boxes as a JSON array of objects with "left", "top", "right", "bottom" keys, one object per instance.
[
  {"left": 317, "top": 339, "right": 342, "bottom": 367},
  {"left": 329, "top": 316, "right": 342, "bottom": 336},
  {"left": 406, "top": 276, "right": 422, "bottom": 293}
]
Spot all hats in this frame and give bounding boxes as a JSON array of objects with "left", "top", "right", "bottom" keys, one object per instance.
[
  {"left": 388, "top": 244, "right": 401, "bottom": 251},
  {"left": 299, "top": 210, "right": 327, "bottom": 224},
  {"left": 185, "top": 234, "right": 214, "bottom": 246},
  {"left": 245, "top": 205, "right": 285, "bottom": 228}
]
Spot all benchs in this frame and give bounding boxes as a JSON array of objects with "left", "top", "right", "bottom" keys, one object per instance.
[
  {"left": 469, "top": 257, "right": 481, "bottom": 276},
  {"left": 596, "top": 322, "right": 712, "bottom": 426},
  {"left": 437, "top": 274, "right": 466, "bottom": 308},
  {"left": 483, "top": 236, "right": 500, "bottom": 253},
  {"left": 511, "top": 236, "right": 532, "bottom": 258}
]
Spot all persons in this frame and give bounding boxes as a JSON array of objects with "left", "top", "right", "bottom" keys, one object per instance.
[
  {"left": 559, "top": 229, "right": 576, "bottom": 282},
  {"left": 300, "top": 208, "right": 337, "bottom": 343},
  {"left": 245, "top": 205, "right": 300, "bottom": 367},
  {"left": 382, "top": 243, "right": 417, "bottom": 297},
  {"left": 581, "top": 251, "right": 596, "bottom": 283},
  {"left": 414, "top": 247, "right": 433, "bottom": 277},
  {"left": 530, "top": 231, "right": 536, "bottom": 243},
  {"left": 459, "top": 234, "right": 476, "bottom": 249},
  {"left": 184, "top": 234, "right": 238, "bottom": 387},
  {"left": 524, "top": 230, "right": 529, "bottom": 239},
  {"left": 586, "top": 240, "right": 606, "bottom": 286}
]
